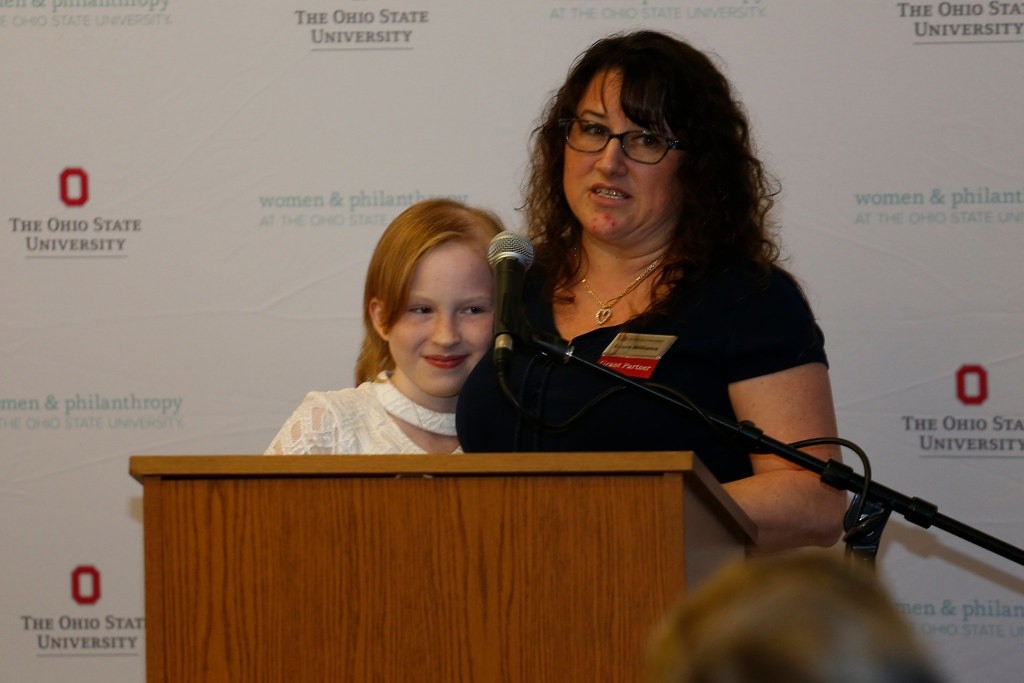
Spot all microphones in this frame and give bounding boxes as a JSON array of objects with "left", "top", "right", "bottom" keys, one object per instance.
[{"left": 485, "top": 230, "right": 535, "bottom": 375}]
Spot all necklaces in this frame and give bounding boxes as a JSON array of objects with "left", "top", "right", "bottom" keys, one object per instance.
[{"left": 574, "top": 245, "right": 662, "bottom": 325}]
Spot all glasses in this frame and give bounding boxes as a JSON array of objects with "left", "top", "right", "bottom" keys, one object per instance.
[{"left": 560, "top": 116, "right": 681, "bottom": 165}]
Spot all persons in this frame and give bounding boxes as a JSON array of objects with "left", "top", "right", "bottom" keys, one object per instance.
[
  {"left": 262, "top": 198, "right": 505, "bottom": 458},
  {"left": 648, "top": 545, "right": 940, "bottom": 683},
  {"left": 454, "top": 29, "right": 847, "bottom": 555}
]
